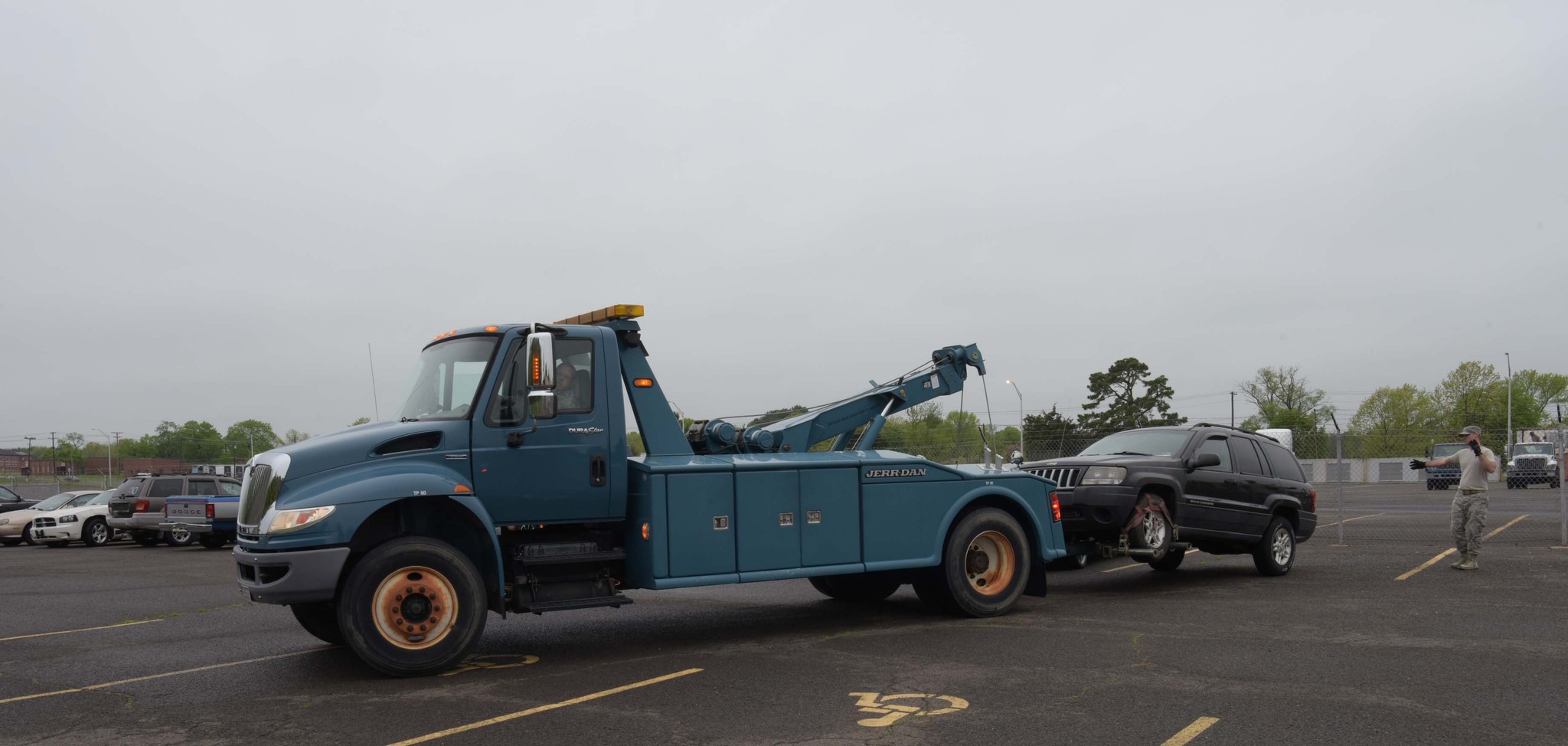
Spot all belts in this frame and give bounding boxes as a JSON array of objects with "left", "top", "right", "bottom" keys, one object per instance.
[{"left": 1458, "top": 489, "right": 1470, "bottom": 495}]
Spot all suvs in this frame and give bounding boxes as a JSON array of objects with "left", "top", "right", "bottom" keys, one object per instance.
[
  {"left": 1507, "top": 442, "right": 1561, "bottom": 489},
  {"left": 1424, "top": 443, "right": 1474, "bottom": 490},
  {"left": 1011, "top": 421, "right": 1314, "bottom": 578},
  {"left": 106, "top": 470, "right": 242, "bottom": 547}
]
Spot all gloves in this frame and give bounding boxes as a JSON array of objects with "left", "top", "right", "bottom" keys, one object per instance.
[
  {"left": 1410, "top": 459, "right": 1426, "bottom": 470},
  {"left": 1469, "top": 439, "right": 1483, "bottom": 456}
]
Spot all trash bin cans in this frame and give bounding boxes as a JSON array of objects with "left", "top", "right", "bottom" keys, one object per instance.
[
  {"left": 57, "top": 466, "right": 66, "bottom": 475},
  {"left": 1556, "top": 453, "right": 1568, "bottom": 482},
  {"left": 21, "top": 467, "right": 30, "bottom": 475}
]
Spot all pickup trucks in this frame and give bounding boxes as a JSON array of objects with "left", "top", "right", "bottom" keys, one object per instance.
[{"left": 157, "top": 495, "right": 240, "bottom": 547}]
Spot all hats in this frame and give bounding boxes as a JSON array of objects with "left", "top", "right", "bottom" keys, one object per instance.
[{"left": 1458, "top": 426, "right": 1481, "bottom": 437}]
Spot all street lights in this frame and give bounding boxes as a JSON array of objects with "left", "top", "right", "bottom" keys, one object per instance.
[
  {"left": 92, "top": 428, "right": 112, "bottom": 488},
  {"left": 1503, "top": 351, "right": 1511, "bottom": 462},
  {"left": 1006, "top": 379, "right": 1023, "bottom": 455},
  {"left": 1325, "top": 408, "right": 1343, "bottom": 544}
]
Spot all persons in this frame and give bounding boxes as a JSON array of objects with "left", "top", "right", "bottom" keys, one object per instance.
[
  {"left": 1409, "top": 425, "right": 1496, "bottom": 570},
  {"left": 499, "top": 362, "right": 581, "bottom": 419}
]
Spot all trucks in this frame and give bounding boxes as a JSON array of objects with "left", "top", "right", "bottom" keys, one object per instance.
[{"left": 231, "top": 302, "right": 1065, "bottom": 675}]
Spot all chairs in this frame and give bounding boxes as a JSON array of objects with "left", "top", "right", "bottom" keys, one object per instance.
[{"left": 571, "top": 369, "right": 592, "bottom": 411}]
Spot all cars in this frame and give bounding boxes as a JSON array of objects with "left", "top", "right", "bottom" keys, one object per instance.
[
  {"left": 0, "top": 490, "right": 105, "bottom": 546},
  {"left": 65, "top": 476, "right": 80, "bottom": 482},
  {"left": 0, "top": 484, "right": 43, "bottom": 513},
  {"left": 30, "top": 488, "right": 134, "bottom": 549}
]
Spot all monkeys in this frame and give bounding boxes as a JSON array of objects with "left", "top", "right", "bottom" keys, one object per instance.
[{"left": 1529, "top": 431, "right": 1542, "bottom": 442}]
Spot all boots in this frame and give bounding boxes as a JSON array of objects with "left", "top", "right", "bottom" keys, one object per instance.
[
  {"left": 1451, "top": 552, "right": 1469, "bottom": 567},
  {"left": 1457, "top": 554, "right": 1478, "bottom": 570}
]
